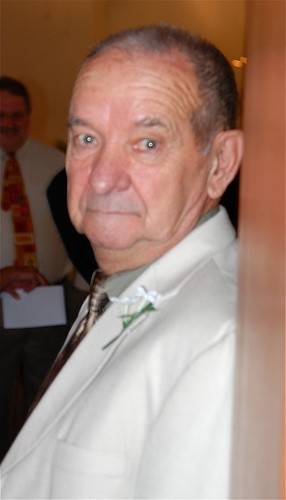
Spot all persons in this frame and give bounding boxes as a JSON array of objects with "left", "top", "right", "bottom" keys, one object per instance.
[
  {"left": 0, "top": 23, "right": 244, "bottom": 500},
  {"left": 0, "top": 76, "right": 77, "bottom": 466}
]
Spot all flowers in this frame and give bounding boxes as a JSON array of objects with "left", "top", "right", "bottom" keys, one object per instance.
[{"left": 102, "top": 285, "right": 161, "bottom": 349}]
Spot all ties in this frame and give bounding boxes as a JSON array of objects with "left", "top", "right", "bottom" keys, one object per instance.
[
  {"left": 27, "top": 270, "right": 112, "bottom": 422},
  {"left": 1, "top": 152, "right": 39, "bottom": 271}
]
